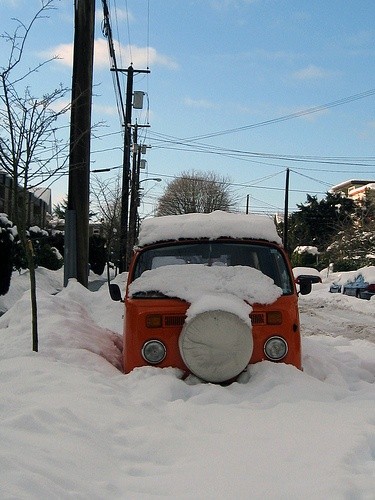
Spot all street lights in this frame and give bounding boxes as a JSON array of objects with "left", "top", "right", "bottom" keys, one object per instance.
[{"left": 127, "top": 177, "right": 162, "bottom": 270}]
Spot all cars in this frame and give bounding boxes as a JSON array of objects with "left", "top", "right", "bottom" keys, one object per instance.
[
  {"left": 296, "top": 274, "right": 322, "bottom": 285},
  {"left": 342, "top": 275, "right": 375, "bottom": 300},
  {"left": 329, "top": 280, "right": 342, "bottom": 293},
  {"left": 108, "top": 236, "right": 312, "bottom": 383}
]
